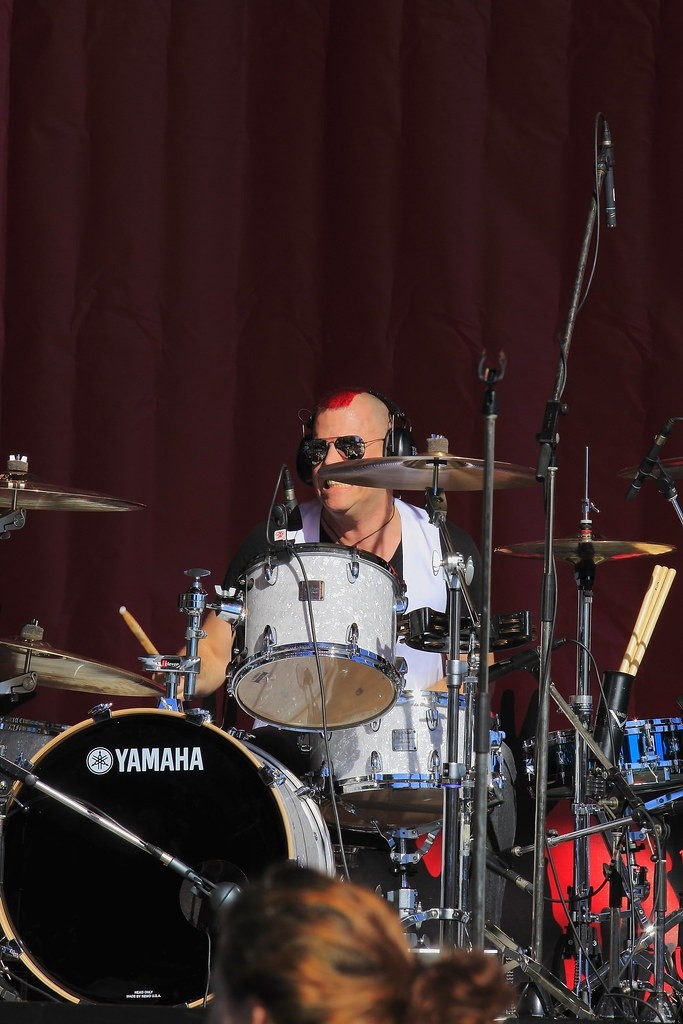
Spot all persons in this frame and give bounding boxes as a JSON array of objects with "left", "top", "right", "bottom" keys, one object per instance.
[
  {"left": 155, "top": 387, "right": 517, "bottom": 947},
  {"left": 211, "top": 866, "right": 514, "bottom": 1024}
]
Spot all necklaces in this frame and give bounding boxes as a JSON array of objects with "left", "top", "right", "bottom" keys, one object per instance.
[{"left": 321, "top": 500, "right": 395, "bottom": 547}]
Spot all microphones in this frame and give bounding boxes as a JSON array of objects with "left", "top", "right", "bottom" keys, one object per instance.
[
  {"left": 626, "top": 420, "right": 676, "bottom": 501},
  {"left": 601, "top": 119, "right": 616, "bottom": 227},
  {"left": 484, "top": 847, "right": 533, "bottom": 896},
  {"left": 211, "top": 884, "right": 243, "bottom": 913},
  {"left": 281, "top": 465, "right": 306, "bottom": 545},
  {"left": 489, "top": 637, "right": 567, "bottom": 682}
]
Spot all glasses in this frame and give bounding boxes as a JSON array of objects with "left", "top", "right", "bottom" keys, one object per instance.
[{"left": 301, "top": 434, "right": 386, "bottom": 467}]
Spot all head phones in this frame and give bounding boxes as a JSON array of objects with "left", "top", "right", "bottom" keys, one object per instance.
[{"left": 296, "top": 390, "right": 418, "bottom": 485}]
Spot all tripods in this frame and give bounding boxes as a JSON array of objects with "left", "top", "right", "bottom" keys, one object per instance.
[{"left": 386, "top": 490, "right": 683, "bottom": 1024}]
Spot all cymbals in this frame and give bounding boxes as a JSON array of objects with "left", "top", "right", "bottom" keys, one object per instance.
[
  {"left": 0, "top": 478, "right": 147, "bottom": 513},
  {"left": 397, "top": 606, "right": 537, "bottom": 654},
  {"left": 494, "top": 539, "right": 677, "bottom": 561},
  {"left": 316, "top": 453, "right": 537, "bottom": 492},
  {"left": 0, "top": 637, "right": 168, "bottom": 698}
]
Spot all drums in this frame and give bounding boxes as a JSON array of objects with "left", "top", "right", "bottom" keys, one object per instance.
[
  {"left": 0, "top": 708, "right": 334, "bottom": 1011},
  {"left": 521, "top": 718, "right": 683, "bottom": 800},
  {"left": 225, "top": 542, "right": 406, "bottom": 731},
  {"left": 297, "top": 685, "right": 502, "bottom": 832},
  {"left": 0, "top": 715, "right": 73, "bottom": 763}
]
